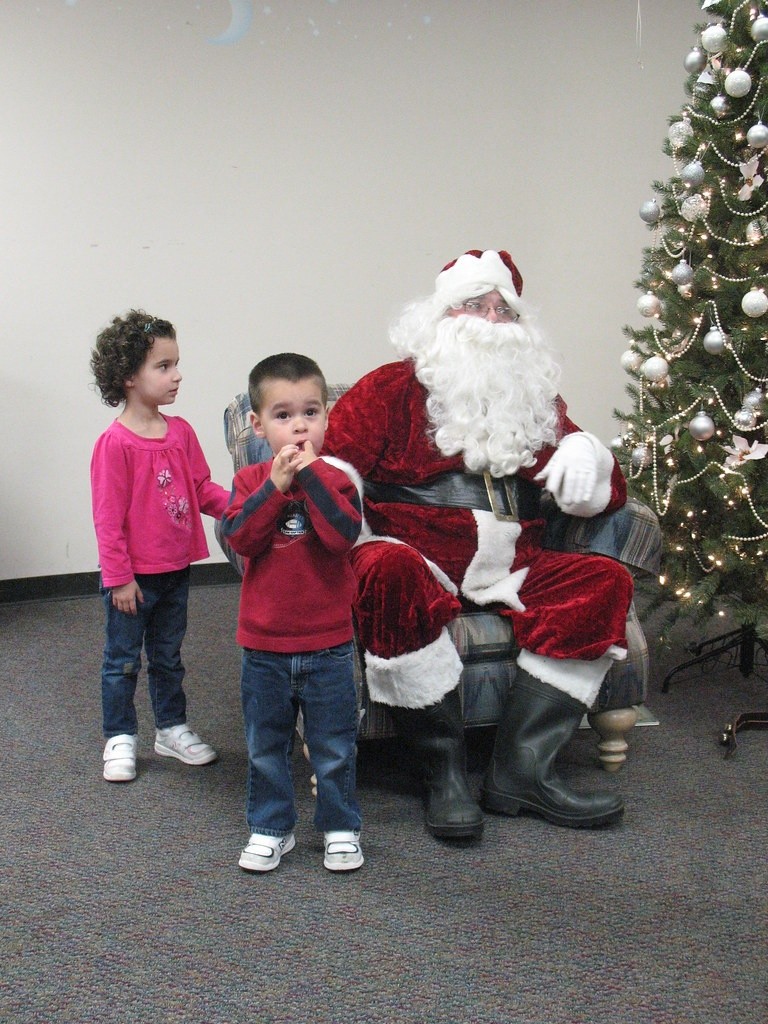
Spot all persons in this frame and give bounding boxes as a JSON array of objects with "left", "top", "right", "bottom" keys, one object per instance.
[
  {"left": 90, "top": 309, "right": 233, "bottom": 783},
  {"left": 219, "top": 351, "right": 364, "bottom": 872},
  {"left": 319, "top": 248, "right": 635, "bottom": 838}
]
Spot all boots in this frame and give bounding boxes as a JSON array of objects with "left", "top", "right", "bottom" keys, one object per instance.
[
  {"left": 396, "top": 685, "right": 485, "bottom": 837},
  {"left": 478, "top": 665, "right": 624, "bottom": 829}
]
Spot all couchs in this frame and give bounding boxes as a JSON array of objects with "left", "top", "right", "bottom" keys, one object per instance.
[{"left": 217, "top": 383, "right": 667, "bottom": 797}]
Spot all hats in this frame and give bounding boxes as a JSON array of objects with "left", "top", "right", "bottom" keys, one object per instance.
[{"left": 434, "top": 248, "right": 524, "bottom": 314}]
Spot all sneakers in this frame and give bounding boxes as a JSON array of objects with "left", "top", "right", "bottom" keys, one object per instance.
[
  {"left": 154, "top": 725, "right": 217, "bottom": 766},
  {"left": 238, "top": 832, "right": 295, "bottom": 871},
  {"left": 103, "top": 734, "right": 137, "bottom": 782},
  {"left": 323, "top": 829, "right": 365, "bottom": 870}
]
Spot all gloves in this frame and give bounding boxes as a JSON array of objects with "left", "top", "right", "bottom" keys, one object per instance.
[{"left": 532, "top": 434, "right": 598, "bottom": 504}]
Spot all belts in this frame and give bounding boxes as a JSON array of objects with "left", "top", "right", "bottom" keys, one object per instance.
[{"left": 360, "top": 471, "right": 559, "bottom": 522}]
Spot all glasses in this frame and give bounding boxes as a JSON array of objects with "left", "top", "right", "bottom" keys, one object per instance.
[{"left": 462, "top": 299, "right": 520, "bottom": 325}]
